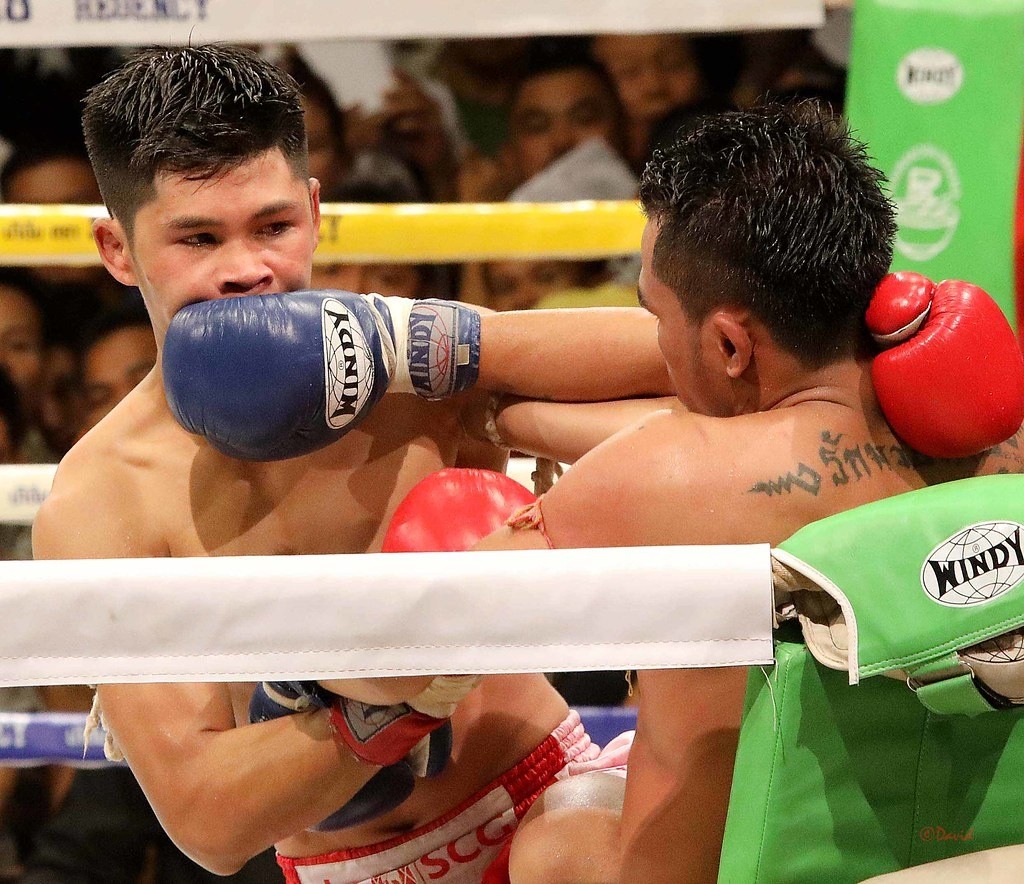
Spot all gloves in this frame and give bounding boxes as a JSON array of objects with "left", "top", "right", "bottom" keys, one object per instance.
[
  {"left": 160, "top": 286, "right": 487, "bottom": 463},
  {"left": 334, "top": 466, "right": 541, "bottom": 767},
  {"left": 861, "top": 267, "right": 1024, "bottom": 454},
  {"left": 247, "top": 680, "right": 450, "bottom": 833}
]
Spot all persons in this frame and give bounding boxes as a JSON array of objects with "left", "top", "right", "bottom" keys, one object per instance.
[
  {"left": 0, "top": 35, "right": 848, "bottom": 883},
  {"left": 163, "top": 91, "right": 1024, "bottom": 884},
  {"left": 29, "top": 52, "right": 1024, "bottom": 883}
]
